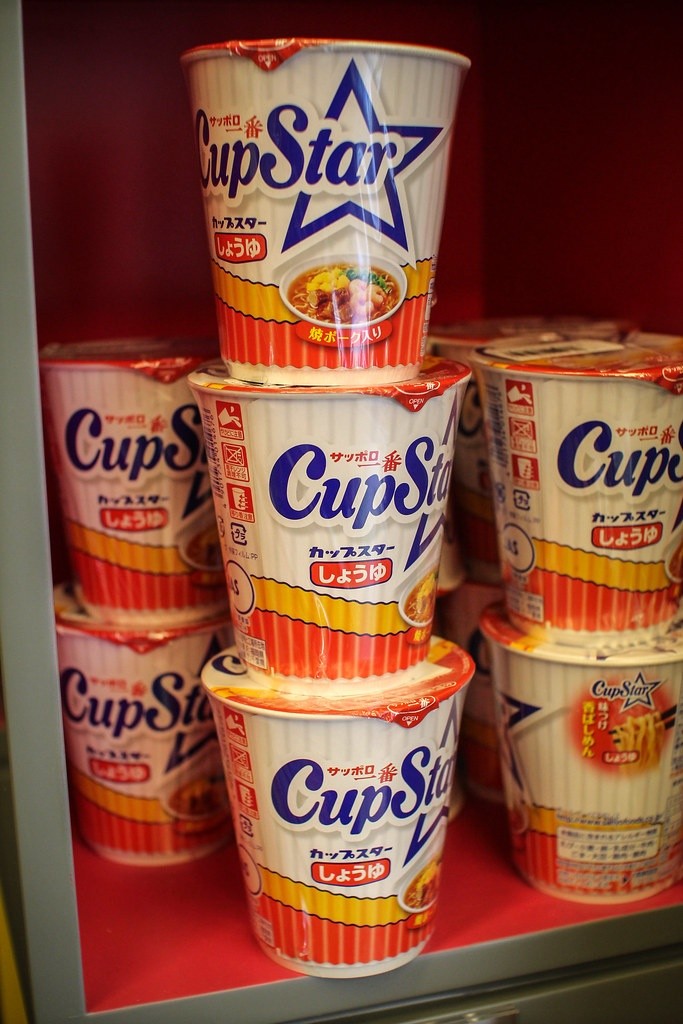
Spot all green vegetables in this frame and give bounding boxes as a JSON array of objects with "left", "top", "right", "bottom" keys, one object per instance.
[{"left": 344, "top": 269, "right": 389, "bottom": 292}]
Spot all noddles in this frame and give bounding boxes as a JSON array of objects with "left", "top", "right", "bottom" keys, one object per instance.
[
  {"left": 289, "top": 264, "right": 398, "bottom": 325},
  {"left": 609, "top": 711, "right": 668, "bottom": 775}
]
